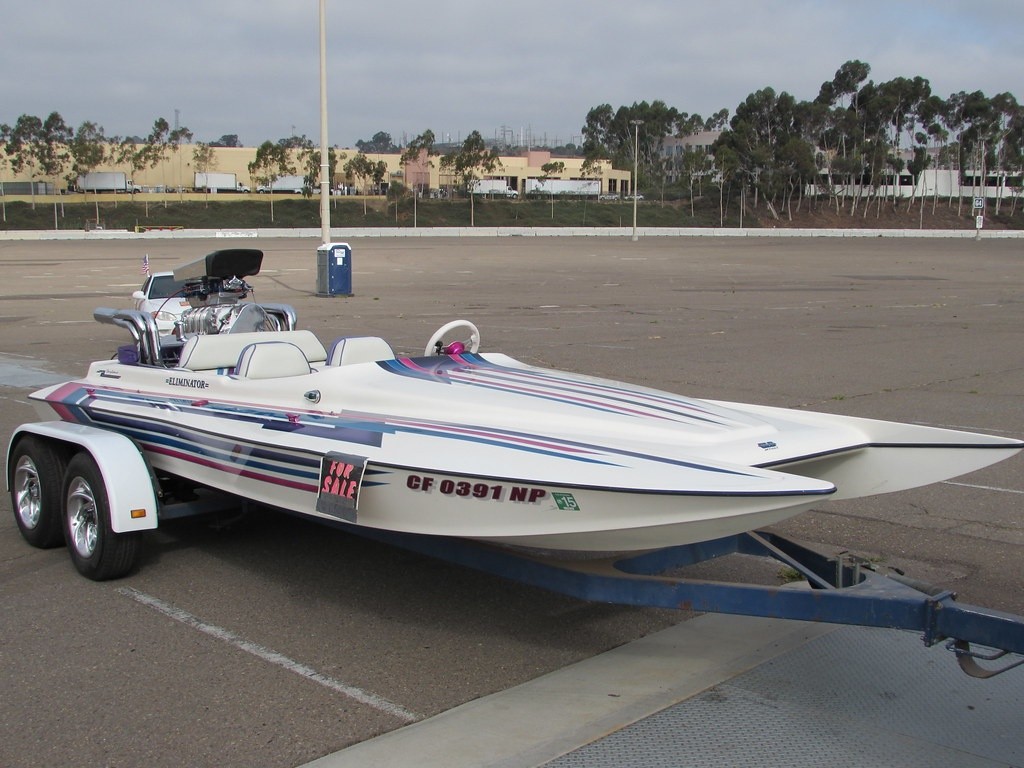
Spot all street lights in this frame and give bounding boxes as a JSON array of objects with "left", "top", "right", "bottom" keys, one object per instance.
[{"left": 630, "top": 118, "right": 645, "bottom": 240}]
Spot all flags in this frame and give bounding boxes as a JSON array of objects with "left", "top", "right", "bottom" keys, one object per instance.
[{"left": 141, "top": 254, "right": 150, "bottom": 275}]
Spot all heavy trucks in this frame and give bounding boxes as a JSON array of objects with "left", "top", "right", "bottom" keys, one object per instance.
[{"left": 522, "top": 178, "right": 601, "bottom": 200}]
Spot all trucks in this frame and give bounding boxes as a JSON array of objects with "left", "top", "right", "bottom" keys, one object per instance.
[
  {"left": 77, "top": 171, "right": 142, "bottom": 194},
  {"left": 465, "top": 179, "right": 519, "bottom": 199},
  {"left": 256, "top": 176, "right": 309, "bottom": 194},
  {"left": 194, "top": 172, "right": 251, "bottom": 193}
]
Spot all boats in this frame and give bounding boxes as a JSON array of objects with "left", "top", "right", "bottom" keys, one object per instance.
[{"left": 31, "top": 245, "right": 1022, "bottom": 563}]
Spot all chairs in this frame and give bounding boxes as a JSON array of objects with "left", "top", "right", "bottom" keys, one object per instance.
[
  {"left": 325, "top": 335, "right": 394, "bottom": 367},
  {"left": 235, "top": 341, "right": 311, "bottom": 380}
]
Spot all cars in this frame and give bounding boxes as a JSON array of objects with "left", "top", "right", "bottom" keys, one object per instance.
[
  {"left": 625, "top": 194, "right": 644, "bottom": 201},
  {"left": 599, "top": 193, "right": 619, "bottom": 200},
  {"left": 133, "top": 271, "right": 242, "bottom": 356},
  {"left": 428, "top": 188, "right": 446, "bottom": 200}
]
